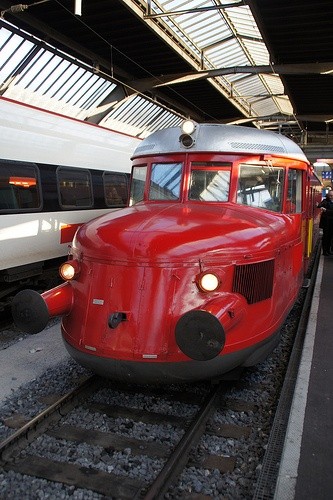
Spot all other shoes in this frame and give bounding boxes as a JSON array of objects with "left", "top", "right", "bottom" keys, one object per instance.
[{"left": 323, "top": 252, "right": 333, "bottom": 255}]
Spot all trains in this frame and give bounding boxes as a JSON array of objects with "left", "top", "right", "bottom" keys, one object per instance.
[
  {"left": 0, "top": 102, "right": 143, "bottom": 329},
  {"left": 13, "top": 117, "right": 325, "bottom": 386}
]
